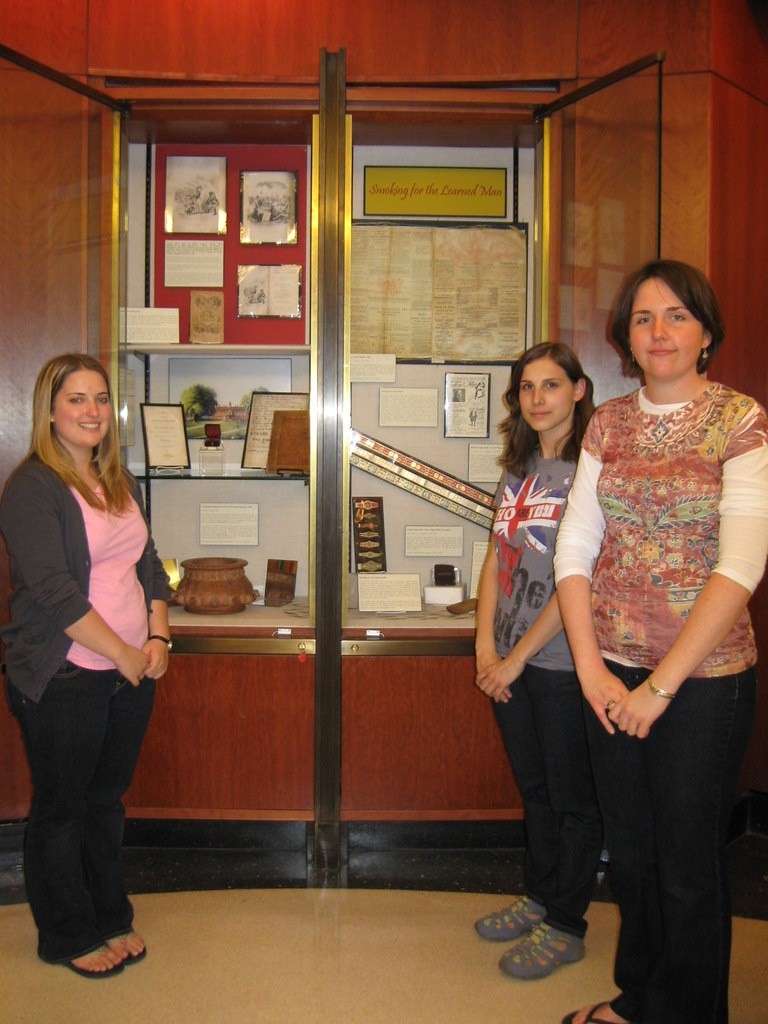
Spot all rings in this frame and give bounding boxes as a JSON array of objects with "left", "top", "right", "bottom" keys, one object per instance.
[{"left": 608, "top": 700, "right": 614, "bottom": 706}]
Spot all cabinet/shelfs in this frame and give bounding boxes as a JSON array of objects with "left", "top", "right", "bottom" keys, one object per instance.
[{"left": 116, "top": 98, "right": 562, "bottom": 821}]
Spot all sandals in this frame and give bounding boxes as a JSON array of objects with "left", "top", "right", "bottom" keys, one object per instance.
[
  {"left": 498, "top": 922, "right": 585, "bottom": 979},
  {"left": 64, "top": 945, "right": 124, "bottom": 978},
  {"left": 561, "top": 1001, "right": 616, "bottom": 1024},
  {"left": 104, "top": 932, "right": 146, "bottom": 964},
  {"left": 475, "top": 896, "right": 548, "bottom": 942}
]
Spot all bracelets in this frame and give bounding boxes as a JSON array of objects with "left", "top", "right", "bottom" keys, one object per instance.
[{"left": 649, "top": 678, "right": 674, "bottom": 698}]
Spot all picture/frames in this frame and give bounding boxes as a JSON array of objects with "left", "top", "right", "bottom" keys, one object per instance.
[
  {"left": 241, "top": 390, "right": 308, "bottom": 470},
  {"left": 443, "top": 372, "right": 492, "bottom": 439},
  {"left": 266, "top": 410, "right": 309, "bottom": 472},
  {"left": 162, "top": 239, "right": 225, "bottom": 289},
  {"left": 162, "top": 155, "right": 228, "bottom": 235},
  {"left": 236, "top": 263, "right": 304, "bottom": 320},
  {"left": 189, "top": 291, "right": 225, "bottom": 345},
  {"left": 239, "top": 169, "right": 298, "bottom": 246},
  {"left": 141, "top": 404, "right": 191, "bottom": 470}
]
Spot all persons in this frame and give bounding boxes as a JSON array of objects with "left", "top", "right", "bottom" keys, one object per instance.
[
  {"left": 553, "top": 259, "right": 768, "bottom": 1024},
  {"left": 474, "top": 341, "right": 597, "bottom": 980},
  {"left": 0, "top": 352, "right": 173, "bottom": 978}
]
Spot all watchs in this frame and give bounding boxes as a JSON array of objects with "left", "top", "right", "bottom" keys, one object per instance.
[{"left": 150, "top": 635, "right": 172, "bottom": 651}]
